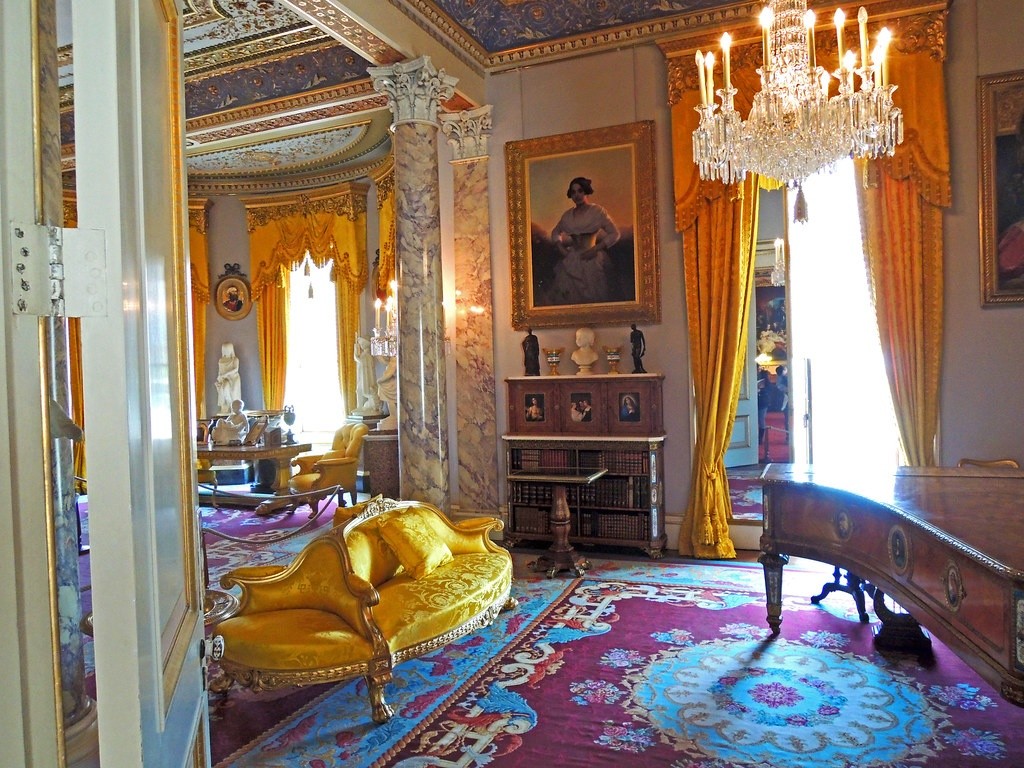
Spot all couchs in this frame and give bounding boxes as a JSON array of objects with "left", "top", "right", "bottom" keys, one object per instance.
[
  {"left": 210, "top": 498, "right": 519, "bottom": 725},
  {"left": 285, "top": 423, "right": 369, "bottom": 518}
]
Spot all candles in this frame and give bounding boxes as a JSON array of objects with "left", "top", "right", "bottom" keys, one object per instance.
[
  {"left": 374, "top": 298, "right": 382, "bottom": 330},
  {"left": 386, "top": 280, "right": 398, "bottom": 329}
]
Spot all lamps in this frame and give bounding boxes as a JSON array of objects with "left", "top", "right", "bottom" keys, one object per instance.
[{"left": 692, "top": 0, "right": 904, "bottom": 224}]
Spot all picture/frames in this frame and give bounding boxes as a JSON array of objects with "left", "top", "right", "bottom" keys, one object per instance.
[
  {"left": 976, "top": 69, "right": 1024, "bottom": 309},
  {"left": 215, "top": 262, "right": 253, "bottom": 321},
  {"left": 755, "top": 276, "right": 788, "bottom": 366},
  {"left": 504, "top": 120, "right": 662, "bottom": 330}
]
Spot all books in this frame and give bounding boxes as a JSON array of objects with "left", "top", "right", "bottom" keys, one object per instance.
[{"left": 510, "top": 448, "right": 650, "bottom": 540}]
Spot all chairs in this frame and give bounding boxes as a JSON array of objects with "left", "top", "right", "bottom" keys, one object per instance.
[
  {"left": 197, "top": 423, "right": 208, "bottom": 445},
  {"left": 957, "top": 458, "right": 1020, "bottom": 469}
]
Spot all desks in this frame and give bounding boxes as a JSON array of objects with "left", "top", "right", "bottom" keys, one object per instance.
[
  {"left": 197, "top": 441, "right": 312, "bottom": 515},
  {"left": 507, "top": 467, "right": 608, "bottom": 580},
  {"left": 759, "top": 462, "right": 1024, "bottom": 709}
]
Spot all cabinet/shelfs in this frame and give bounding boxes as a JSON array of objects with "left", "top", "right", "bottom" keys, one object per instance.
[{"left": 501, "top": 373, "right": 668, "bottom": 560}]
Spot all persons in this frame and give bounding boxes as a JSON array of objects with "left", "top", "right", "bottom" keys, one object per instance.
[
  {"left": 215, "top": 343, "right": 244, "bottom": 414},
  {"left": 570, "top": 326, "right": 600, "bottom": 367},
  {"left": 370, "top": 336, "right": 398, "bottom": 422},
  {"left": 351, "top": 334, "right": 380, "bottom": 416},
  {"left": 212, "top": 400, "right": 248, "bottom": 446},
  {"left": 751, "top": 297, "right": 792, "bottom": 450},
  {"left": 628, "top": 322, "right": 650, "bottom": 375},
  {"left": 521, "top": 327, "right": 542, "bottom": 375}
]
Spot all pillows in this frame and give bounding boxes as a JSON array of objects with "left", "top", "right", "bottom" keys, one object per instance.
[
  {"left": 317, "top": 449, "right": 346, "bottom": 475},
  {"left": 334, "top": 494, "right": 383, "bottom": 528},
  {"left": 377, "top": 506, "right": 454, "bottom": 580}
]
